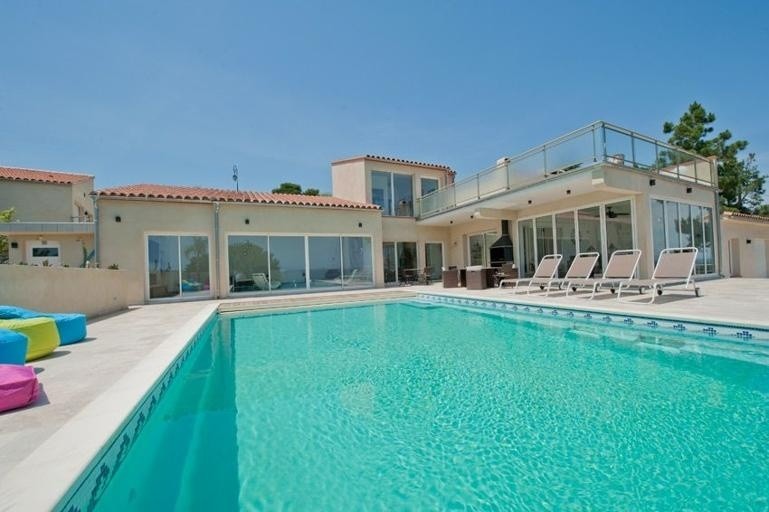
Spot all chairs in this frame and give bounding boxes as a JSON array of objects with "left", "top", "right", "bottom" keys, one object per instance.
[
  {"left": 315, "top": 268, "right": 359, "bottom": 286},
  {"left": 251, "top": 272, "right": 281, "bottom": 290},
  {"left": 422, "top": 266, "right": 433, "bottom": 285},
  {"left": 499, "top": 246, "right": 701, "bottom": 305},
  {"left": 182, "top": 279, "right": 203, "bottom": 292}
]
